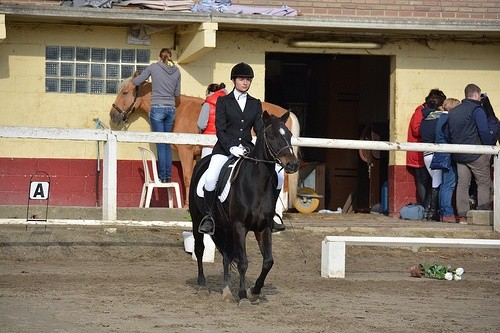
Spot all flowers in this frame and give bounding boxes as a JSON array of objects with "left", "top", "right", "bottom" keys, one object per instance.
[{"left": 419, "top": 263, "right": 465, "bottom": 281}]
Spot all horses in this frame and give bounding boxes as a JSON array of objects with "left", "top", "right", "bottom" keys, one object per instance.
[
  {"left": 188, "top": 108, "right": 301, "bottom": 309},
  {"left": 110, "top": 70, "right": 300, "bottom": 208}
]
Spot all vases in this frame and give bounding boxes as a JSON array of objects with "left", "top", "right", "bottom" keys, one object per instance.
[{"left": 411, "top": 263, "right": 426, "bottom": 279}]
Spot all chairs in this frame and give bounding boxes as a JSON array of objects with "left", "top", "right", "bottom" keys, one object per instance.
[{"left": 138, "top": 146, "right": 182, "bottom": 209}]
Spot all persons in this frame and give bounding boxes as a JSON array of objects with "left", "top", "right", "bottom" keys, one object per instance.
[
  {"left": 406, "top": 84, "right": 498, "bottom": 326},
  {"left": 132, "top": 48, "right": 181, "bottom": 183},
  {"left": 196, "top": 83, "right": 229, "bottom": 160},
  {"left": 200, "top": 62, "right": 285, "bottom": 233}
]
